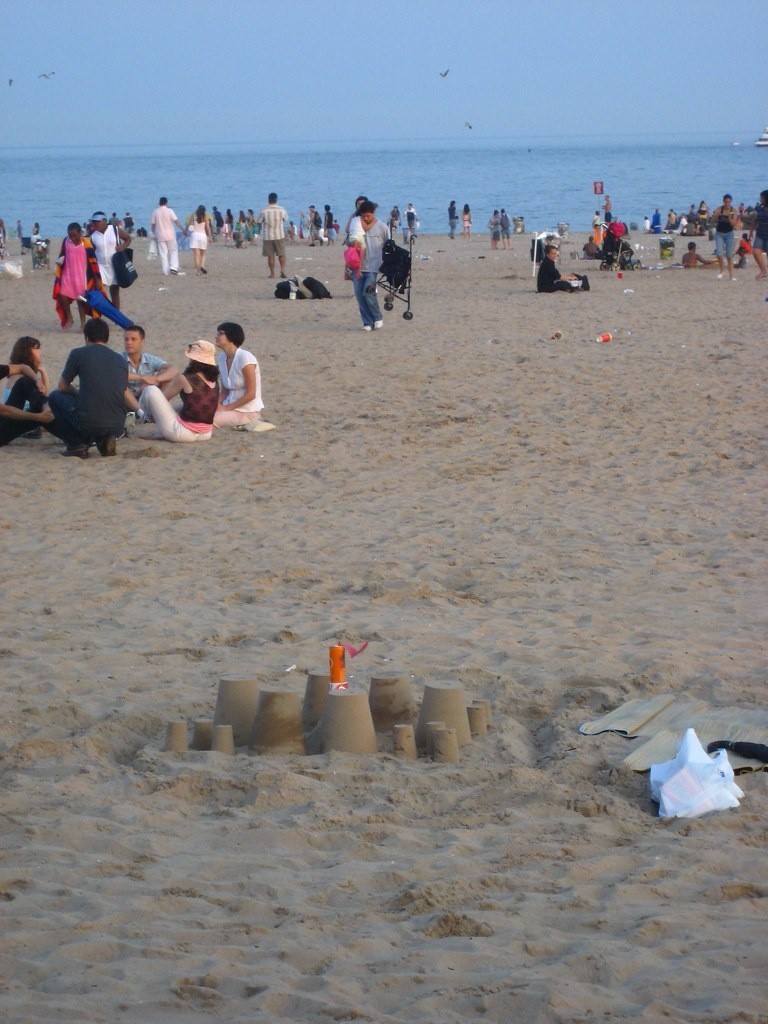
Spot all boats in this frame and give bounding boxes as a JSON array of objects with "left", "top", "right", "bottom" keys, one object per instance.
[{"left": 754, "top": 125, "right": 768, "bottom": 148}]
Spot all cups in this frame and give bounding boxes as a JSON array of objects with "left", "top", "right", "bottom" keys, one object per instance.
[
  {"left": 618, "top": 272, "right": 623, "bottom": 280},
  {"left": 595, "top": 333, "right": 613, "bottom": 343}
]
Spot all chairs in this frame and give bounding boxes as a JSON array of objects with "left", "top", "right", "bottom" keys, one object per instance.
[
  {"left": 534, "top": 229, "right": 564, "bottom": 266},
  {"left": 20, "top": 236, "right": 33, "bottom": 255}
]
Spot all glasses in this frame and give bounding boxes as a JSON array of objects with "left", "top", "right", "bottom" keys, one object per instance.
[{"left": 217, "top": 332, "right": 225, "bottom": 336}]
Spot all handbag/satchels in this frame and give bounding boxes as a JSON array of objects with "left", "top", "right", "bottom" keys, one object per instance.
[
  {"left": 274, "top": 276, "right": 332, "bottom": 300},
  {"left": 113, "top": 248, "right": 138, "bottom": 288},
  {"left": 649, "top": 728, "right": 745, "bottom": 818},
  {"left": 234, "top": 420, "right": 276, "bottom": 432}
]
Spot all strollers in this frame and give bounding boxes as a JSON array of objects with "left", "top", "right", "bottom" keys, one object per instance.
[
  {"left": 365, "top": 217, "right": 417, "bottom": 320},
  {"left": 599, "top": 221, "right": 643, "bottom": 272}
]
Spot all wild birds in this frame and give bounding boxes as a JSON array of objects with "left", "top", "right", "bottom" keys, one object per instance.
[
  {"left": 37, "top": 71, "right": 56, "bottom": 80},
  {"left": 8, "top": 78, "right": 14, "bottom": 86},
  {"left": 439, "top": 68, "right": 451, "bottom": 79},
  {"left": 463, "top": 121, "right": 472, "bottom": 130}
]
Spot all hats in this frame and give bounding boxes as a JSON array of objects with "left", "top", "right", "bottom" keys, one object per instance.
[
  {"left": 89, "top": 214, "right": 107, "bottom": 220},
  {"left": 185, "top": 340, "right": 217, "bottom": 366}
]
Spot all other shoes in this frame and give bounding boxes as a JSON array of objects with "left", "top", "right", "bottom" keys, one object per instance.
[
  {"left": 362, "top": 319, "right": 383, "bottom": 331},
  {"left": 200, "top": 266, "right": 207, "bottom": 274},
  {"left": 61, "top": 449, "right": 90, "bottom": 459},
  {"left": 170, "top": 269, "right": 178, "bottom": 274},
  {"left": 101, "top": 433, "right": 116, "bottom": 456},
  {"left": 20, "top": 427, "right": 42, "bottom": 439}
]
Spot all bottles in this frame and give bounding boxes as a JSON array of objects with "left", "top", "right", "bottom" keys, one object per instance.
[{"left": 289, "top": 287, "right": 296, "bottom": 300}]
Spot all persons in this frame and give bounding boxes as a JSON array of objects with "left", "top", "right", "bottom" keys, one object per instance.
[
  {"left": 4, "top": 335, "right": 55, "bottom": 431},
  {"left": 343, "top": 201, "right": 391, "bottom": 330},
  {"left": 48, "top": 318, "right": 130, "bottom": 459},
  {"left": 114, "top": 325, "right": 179, "bottom": 419},
  {"left": 90, "top": 211, "right": 133, "bottom": 311},
  {"left": 52, "top": 222, "right": 106, "bottom": 334},
  {"left": 211, "top": 206, "right": 224, "bottom": 234},
  {"left": 257, "top": 192, "right": 288, "bottom": 278},
  {"left": 123, "top": 212, "right": 135, "bottom": 234},
  {"left": 308, "top": 205, "right": 324, "bottom": 247},
  {"left": 224, "top": 208, "right": 234, "bottom": 248},
  {"left": 212, "top": 323, "right": 264, "bottom": 430},
  {"left": 289, "top": 221, "right": 297, "bottom": 241},
  {"left": 0, "top": 363, "right": 55, "bottom": 448},
  {"left": 236, "top": 208, "right": 257, "bottom": 249},
  {"left": 643, "top": 189, "right": 768, "bottom": 281},
  {"left": 447, "top": 200, "right": 473, "bottom": 240},
  {"left": 324, "top": 204, "right": 336, "bottom": 247},
  {"left": 488, "top": 208, "right": 513, "bottom": 251},
  {"left": 536, "top": 244, "right": 581, "bottom": 293},
  {"left": 181, "top": 204, "right": 211, "bottom": 276},
  {"left": 139, "top": 340, "right": 220, "bottom": 443},
  {"left": 343, "top": 210, "right": 377, "bottom": 280},
  {"left": 345, "top": 196, "right": 369, "bottom": 232},
  {"left": 580, "top": 195, "right": 613, "bottom": 258},
  {"left": 403, "top": 202, "right": 420, "bottom": 235},
  {"left": 108, "top": 212, "right": 122, "bottom": 227},
  {"left": 386, "top": 205, "right": 401, "bottom": 240},
  {"left": 151, "top": 196, "right": 188, "bottom": 276}
]
